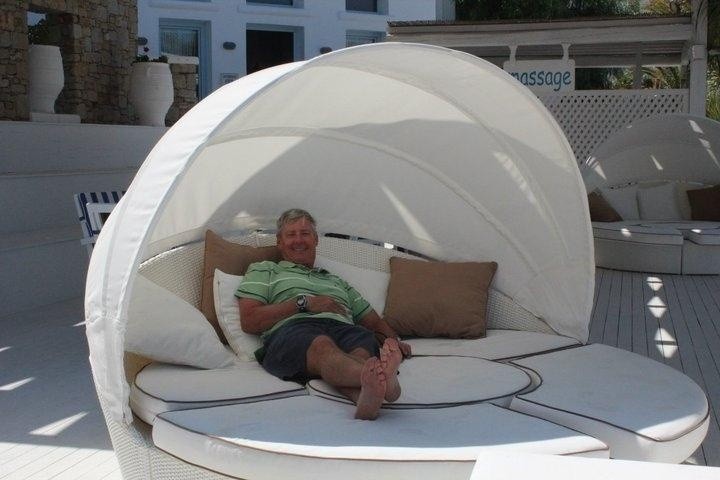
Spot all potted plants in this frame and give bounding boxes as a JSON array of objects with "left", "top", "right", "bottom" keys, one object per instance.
[
  {"left": 128, "top": 54, "right": 176, "bottom": 126},
  {"left": 28, "top": 17, "right": 66, "bottom": 113}
]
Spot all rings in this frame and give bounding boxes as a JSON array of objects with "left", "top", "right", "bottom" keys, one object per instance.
[{"left": 407, "top": 352, "right": 411, "bottom": 355}]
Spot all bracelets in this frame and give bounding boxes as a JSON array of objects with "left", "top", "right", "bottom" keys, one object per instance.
[{"left": 393, "top": 335, "right": 403, "bottom": 342}]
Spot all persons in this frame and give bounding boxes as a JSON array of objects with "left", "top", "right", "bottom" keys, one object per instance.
[{"left": 234, "top": 208, "right": 412, "bottom": 419}]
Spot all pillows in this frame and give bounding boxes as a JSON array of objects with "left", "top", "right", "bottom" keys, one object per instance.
[{"left": 586, "top": 180, "right": 719, "bottom": 224}]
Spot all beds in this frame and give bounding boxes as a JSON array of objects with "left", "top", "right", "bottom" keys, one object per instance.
[
  {"left": 124, "top": 232, "right": 711, "bottom": 480},
  {"left": 581, "top": 180, "right": 719, "bottom": 275}
]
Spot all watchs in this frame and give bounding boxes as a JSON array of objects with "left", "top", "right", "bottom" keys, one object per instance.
[{"left": 295, "top": 294, "right": 308, "bottom": 312}]
